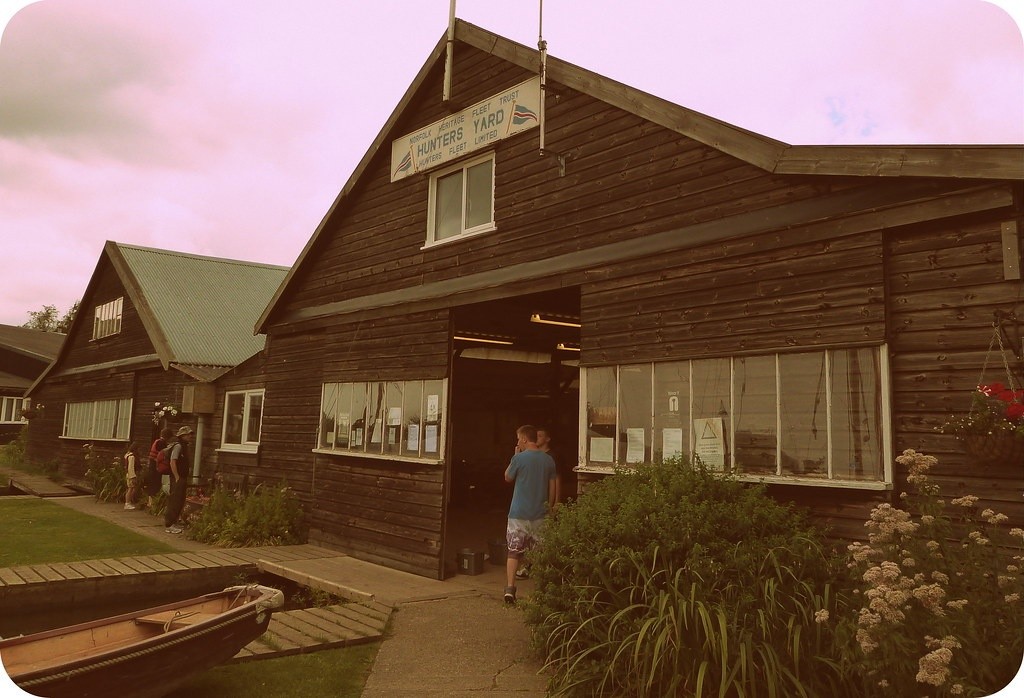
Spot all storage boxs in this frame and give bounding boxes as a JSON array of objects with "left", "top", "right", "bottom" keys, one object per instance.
[
  {"left": 488, "top": 539, "right": 508, "bottom": 565},
  {"left": 456, "top": 549, "right": 485, "bottom": 576}
]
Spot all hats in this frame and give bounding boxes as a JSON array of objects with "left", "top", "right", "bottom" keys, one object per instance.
[{"left": 175, "top": 425, "right": 193, "bottom": 437}]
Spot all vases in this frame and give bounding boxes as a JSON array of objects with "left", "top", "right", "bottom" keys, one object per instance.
[{"left": 957, "top": 427, "right": 1024, "bottom": 464}]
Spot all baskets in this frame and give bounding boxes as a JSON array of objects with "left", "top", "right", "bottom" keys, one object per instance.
[{"left": 964, "top": 433, "right": 1024, "bottom": 464}]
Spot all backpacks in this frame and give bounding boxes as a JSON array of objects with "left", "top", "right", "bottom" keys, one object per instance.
[
  {"left": 156, "top": 442, "right": 181, "bottom": 476},
  {"left": 125, "top": 453, "right": 143, "bottom": 473},
  {"left": 149, "top": 438, "right": 167, "bottom": 473}
]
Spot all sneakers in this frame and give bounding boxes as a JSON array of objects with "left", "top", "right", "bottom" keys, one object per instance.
[
  {"left": 503, "top": 586, "right": 517, "bottom": 603},
  {"left": 124, "top": 505, "right": 135, "bottom": 510},
  {"left": 515, "top": 568, "right": 529, "bottom": 580},
  {"left": 165, "top": 524, "right": 182, "bottom": 534}
]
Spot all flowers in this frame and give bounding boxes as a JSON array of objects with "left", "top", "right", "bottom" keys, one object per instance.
[{"left": 930, "top": 382, "right": 1024, "bottom": 443}]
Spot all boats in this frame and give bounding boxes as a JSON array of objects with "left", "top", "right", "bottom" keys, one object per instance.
[{"left": 0, "top": 581, "right": 285, "bottom": 697}]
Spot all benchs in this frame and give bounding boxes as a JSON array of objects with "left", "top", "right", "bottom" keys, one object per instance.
[{"left": 135, "top": 610, "right": 222, "bottom": 628}]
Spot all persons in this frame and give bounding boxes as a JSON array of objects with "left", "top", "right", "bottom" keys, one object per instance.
[
  {"left": 515, "top": 427, "right": 561, "bottom": 580},
  {"left": 165, "top": 426, "right": 195, "bottom": 534},
  {"left": 505, "top": 425, "right": 557, "bottom": 603},
  {"left": 147, "top": 427, "right": 173, "bottom": 506},
  {"left": 124, "top": 442, "right": 144, "bottom": 509}
]
